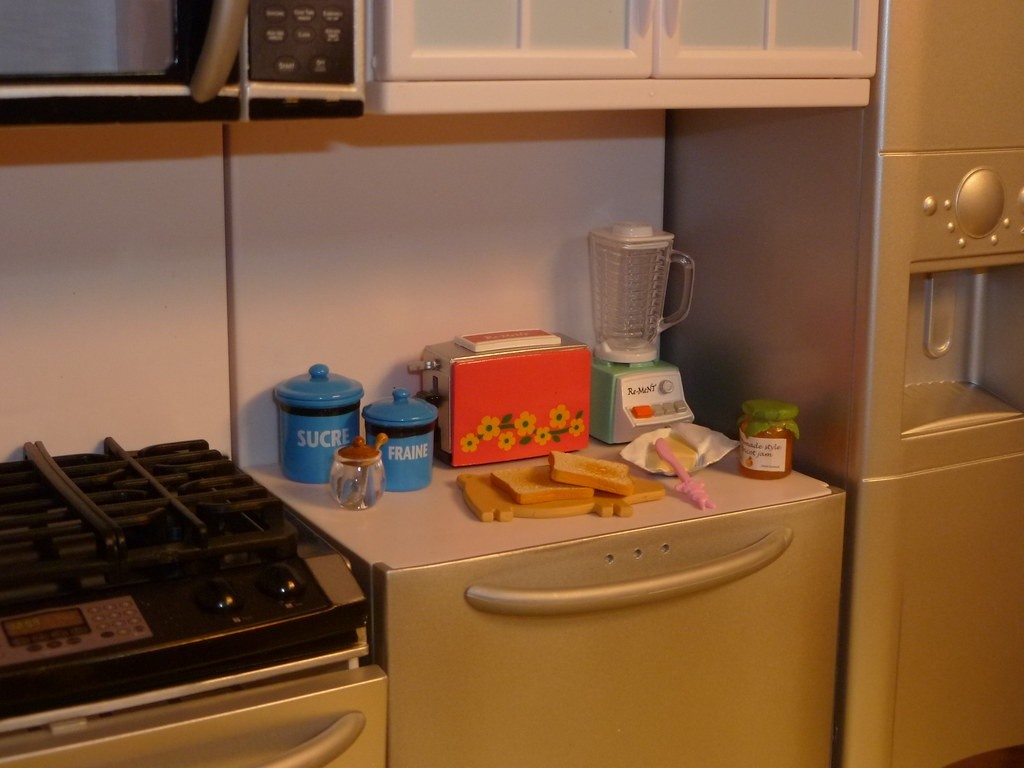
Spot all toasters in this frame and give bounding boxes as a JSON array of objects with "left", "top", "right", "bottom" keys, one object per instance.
[{"left": 409, "top": 329, "right": 591, "bottom": 468}]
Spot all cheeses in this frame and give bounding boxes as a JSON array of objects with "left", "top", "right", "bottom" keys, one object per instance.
[{"left": 648, "top": 436, "right": 698, "bottom": 471}]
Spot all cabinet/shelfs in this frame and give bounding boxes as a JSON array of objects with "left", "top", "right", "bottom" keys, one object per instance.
[
  {"left": 241, "top": 432, "right": 846, "bottom": 768},
  {"left": 365, "top": 0, "right": 883, "bottom": 117},
  {"left": 0, "top": 640, "right": 388, "bottom": 768}
]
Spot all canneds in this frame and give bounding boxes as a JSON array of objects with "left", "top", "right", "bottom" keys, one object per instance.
[{"left": 738, "top": 398, "right": 801, "bottom": 481}]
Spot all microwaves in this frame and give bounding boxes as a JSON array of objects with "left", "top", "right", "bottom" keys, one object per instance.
[{"left": 0, "top": 0, "right": 367, "bottom": 125}]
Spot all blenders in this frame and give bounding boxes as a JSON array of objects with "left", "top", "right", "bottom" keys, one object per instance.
[{"left": 590, "top": 222, "right": 695, "bottom": 444}]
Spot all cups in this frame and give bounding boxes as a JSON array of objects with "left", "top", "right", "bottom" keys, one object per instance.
[
  {"left": 330, "top": 433, "right": 388, "bottom": 512},
  {"left": 361, "top": 388, "right": 438, "bottom": 493},
  {"left": 274, "top": 363, "right": 365, "bottom": 484}
]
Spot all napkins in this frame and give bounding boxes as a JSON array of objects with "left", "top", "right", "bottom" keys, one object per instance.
[{"left": 620, "top": 422, "right": 740, "bottom": 476}]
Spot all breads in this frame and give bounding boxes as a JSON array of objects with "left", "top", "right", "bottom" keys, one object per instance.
[{"left": 488, "top": 451, "right": 635, "bottom": 504}]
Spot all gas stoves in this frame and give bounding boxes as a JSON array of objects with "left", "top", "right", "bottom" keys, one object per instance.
[{"left": 0, "top": 436, "right": 370, "bottom": 735}]
